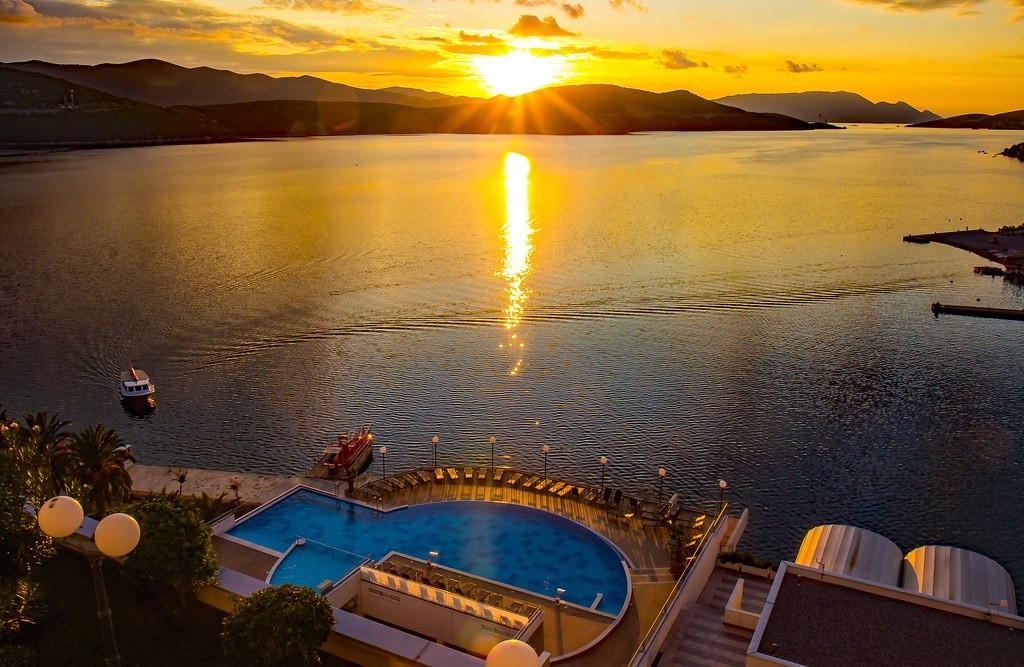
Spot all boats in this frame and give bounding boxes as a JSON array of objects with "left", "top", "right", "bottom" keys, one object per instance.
[
  {"left": 306, "top": 422, "right": 375, "bottom": 485},
  {"left": 118, "top": 365, "right": 156, "bottom": 414}
]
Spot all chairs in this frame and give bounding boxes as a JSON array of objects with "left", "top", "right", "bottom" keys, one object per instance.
[{"left": 358, "top": 463, "right": 709, "bottom": 623}]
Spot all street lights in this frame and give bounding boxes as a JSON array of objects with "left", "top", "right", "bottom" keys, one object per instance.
[
  {"left": 601, "top": 456, "right": 608, "bottom": 492},
  {"left": 432, "top": 436, "right": 439, "bottom": 470},
  {"left": 718, "top": 480, "right": 727, "bottom": 513},
  {"left": 36, "top": 495, "right": 141, "bottom": 667},
  {"left": 658, "top": 468, "right": 666, "bottom": 506},
  {"left": 379, "top": 446, "right": 386, "bottom": 483},
  {"left": 489, "top": 436, "right": 497, "bottom": 471},
  {"left": 543, "top": 444, "right": 549, "bottom": 485}
]
